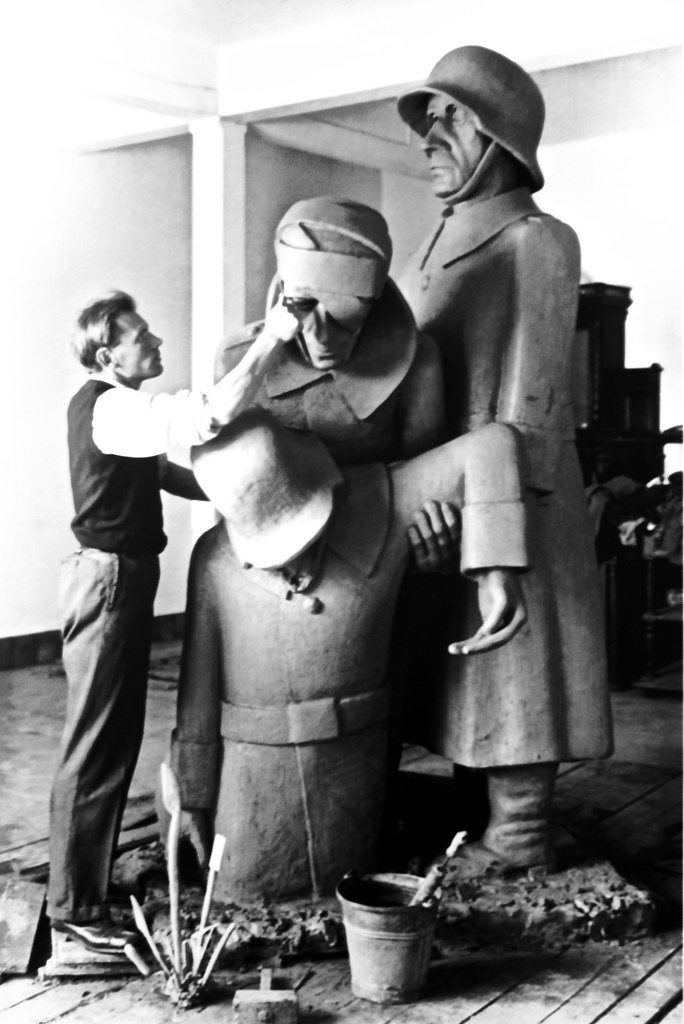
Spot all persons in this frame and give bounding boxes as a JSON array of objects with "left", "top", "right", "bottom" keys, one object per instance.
[
  {"left": 47, "top": 290, "right": 305, "bottom": 956},
  {"left": 212, "top": 197, "right": 460, "bottom": 580},
  {"left": 394, "top": 47, "right": 615, "bottom": 885},
  {"left": 149, "top": 407, "right": 528, "bottom": 894}
]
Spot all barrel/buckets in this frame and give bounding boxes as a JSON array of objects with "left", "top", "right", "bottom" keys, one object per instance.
[{"left": 337, "top": 875, "right": 444, "bottom": 1004}]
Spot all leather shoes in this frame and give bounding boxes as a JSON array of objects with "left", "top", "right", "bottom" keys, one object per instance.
[{"left": 51, "top": 916, "right": 136, "bottom": 953}]
